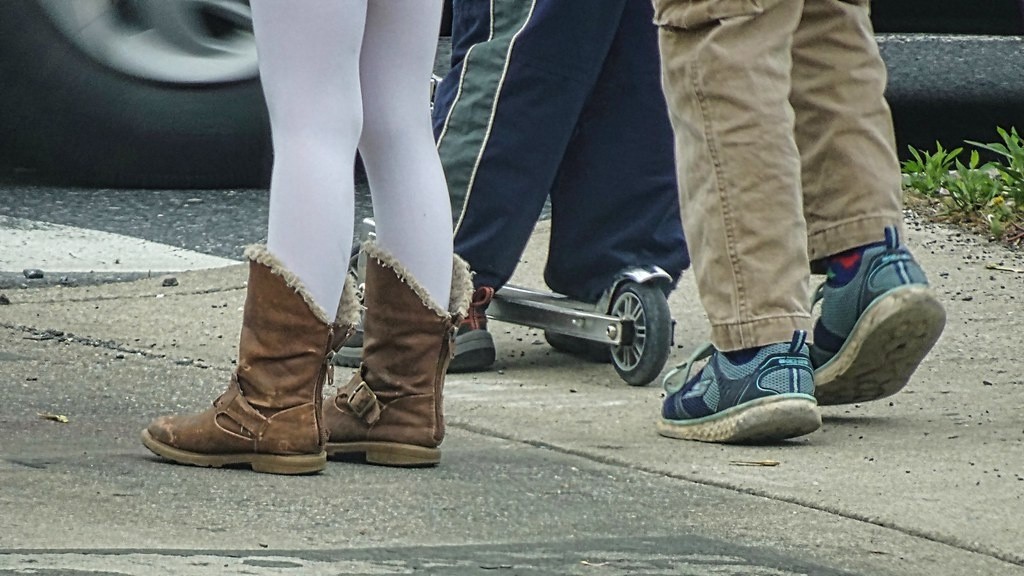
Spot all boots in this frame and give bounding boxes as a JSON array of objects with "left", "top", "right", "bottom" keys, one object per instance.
[
  {"left": 323, "top": 240, "right": 478, "bottom": 466},
  {"left": 140, "top": 241, "right": 368, "bottom": 474}
]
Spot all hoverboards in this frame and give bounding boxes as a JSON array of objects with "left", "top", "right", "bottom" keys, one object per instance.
[{"left": 347, "top": 67, "right": 677, "bottom": 387}]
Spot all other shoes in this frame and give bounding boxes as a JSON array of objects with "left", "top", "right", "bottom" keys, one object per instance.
[
  {"left": 544, "top": 332, "right": 609, "bottom": 363},
  {"left": 331, "top": 288, "right": 496, "bottom": 374}
]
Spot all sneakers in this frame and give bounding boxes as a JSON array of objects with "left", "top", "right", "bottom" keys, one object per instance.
[
  {"left": 808, "top": 227, "right": 946, "bottom": 406},
  {"left": 658, "top": 331, "right": 821, "bottom": 443}
]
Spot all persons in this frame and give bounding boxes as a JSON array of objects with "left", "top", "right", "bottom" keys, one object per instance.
[
  {"left": 139, "top": 0, "right": 459, "bottom": 477},
  {"left": 326, "top": 0, "right": 692, "bottom": 384},
  {"left": 650, "top": 0, "right": 945, "bottom": 447}
]
